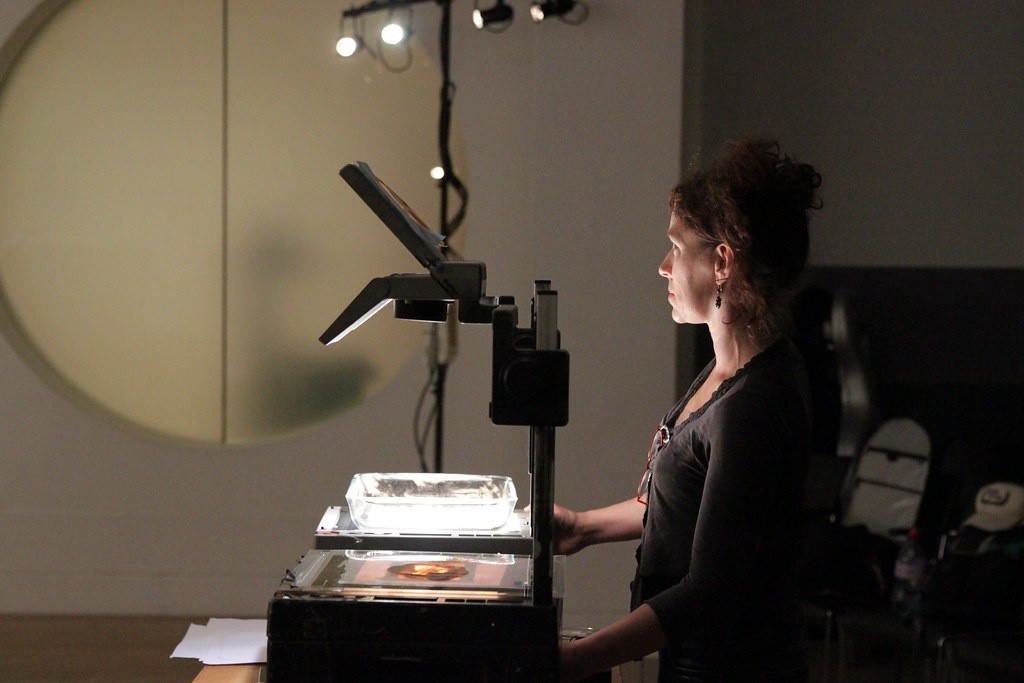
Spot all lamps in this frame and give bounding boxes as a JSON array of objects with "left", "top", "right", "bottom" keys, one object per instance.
[{"left": 335, "top": 0, "right": 591, "bottom": 55}]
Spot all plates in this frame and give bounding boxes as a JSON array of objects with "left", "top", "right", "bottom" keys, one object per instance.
[{"left": 346, "top": 471, "right": 518, "bottom": 532}]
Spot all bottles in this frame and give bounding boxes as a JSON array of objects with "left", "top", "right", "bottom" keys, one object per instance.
[{"left": 891, "top": 527, "right": 927, "bottom": 612}]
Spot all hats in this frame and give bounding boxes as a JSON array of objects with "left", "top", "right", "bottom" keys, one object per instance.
[{"left": 960, "top": 482, "right": 1024, "bottom": 531}]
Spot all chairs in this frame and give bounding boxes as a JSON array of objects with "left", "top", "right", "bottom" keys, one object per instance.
[{"left": 803, "top": 413, "right": 1024, "bottom": 683}]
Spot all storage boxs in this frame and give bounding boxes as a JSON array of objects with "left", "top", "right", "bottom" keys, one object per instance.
[{"left": 345, "top": 472, "right": 519, "bottom": 531}]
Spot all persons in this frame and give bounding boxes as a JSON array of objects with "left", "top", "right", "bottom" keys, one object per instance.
[{"left": 524, "top": 136, "right": 825, "bottom": 683}]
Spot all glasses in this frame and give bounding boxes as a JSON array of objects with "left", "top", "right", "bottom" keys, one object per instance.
[{"left": 637, "top": 425, "right": 670, "bottom": 506}]
